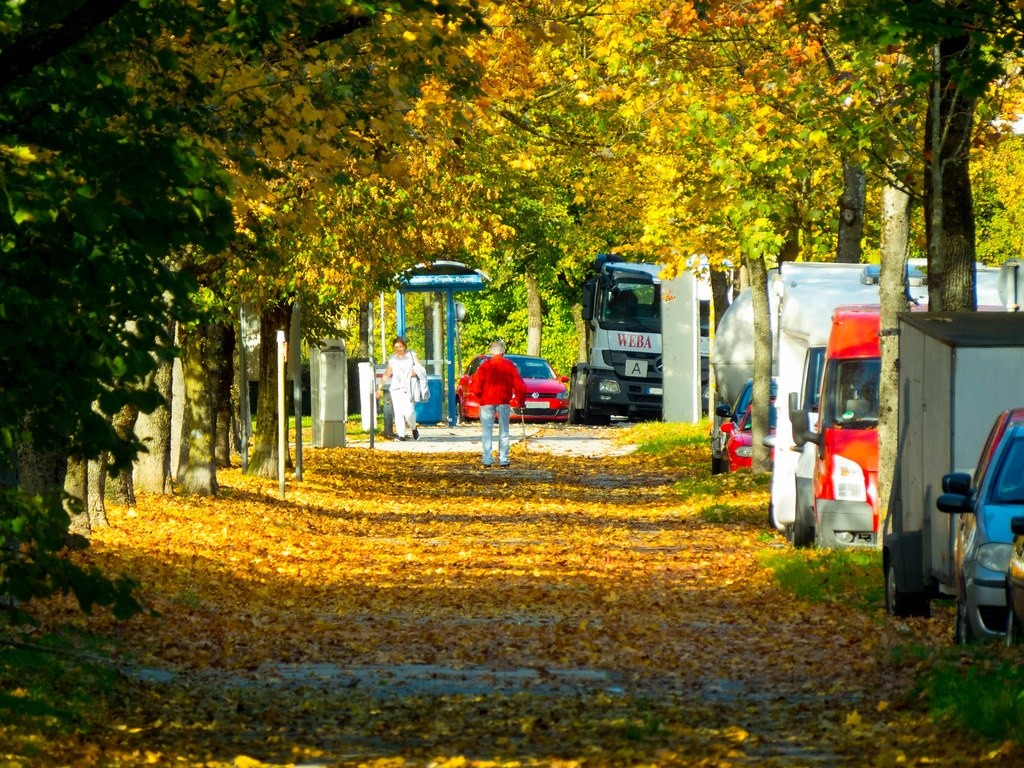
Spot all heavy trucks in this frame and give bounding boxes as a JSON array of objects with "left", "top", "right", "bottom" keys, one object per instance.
[{"left": 570, "top": 254, "right": 733, "bottom": 428}]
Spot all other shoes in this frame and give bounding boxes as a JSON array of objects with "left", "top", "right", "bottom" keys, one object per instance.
[
  {"left": 483, "top": 464, "right": 492, "bottom": 470},
  {"left": 412, "top": 429, "right": 420, "bottom": 441},
  {"left": 500, "top": 460, "right": 511, "bottom": 467},
  {"left": 394, "top": 436, "right": 405, "bottom": 442}
]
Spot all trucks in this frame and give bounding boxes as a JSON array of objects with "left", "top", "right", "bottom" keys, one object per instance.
[
  {"left": 768, "top": 260, "right": 1024, "bottom": 535},
  {"left": 883, "top": 311, "right": 1024, "bottom": 617},
  {"left": 791, "top": 303, "right": 1008, "bottom": 552}
]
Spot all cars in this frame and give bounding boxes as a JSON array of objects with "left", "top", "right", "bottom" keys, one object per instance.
[
  {"left": 720, "top": 396, "right": 777, "bottom": 475},
  {"left": 456, "top": 354, "right": 571, "bottom": 424},
  {"left": 935, "top": 408, "right": 1024, "bottom": 653}
]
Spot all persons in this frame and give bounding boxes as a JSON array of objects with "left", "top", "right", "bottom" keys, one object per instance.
[
  {"left": 376, "top": 337, "right": 424, "bottom": 442},
  {"left": 471, "top": 340, "right": 526, "bottom": 467},
  {"left": 857, "top": 381, "right": 877, "bottom": 410}
]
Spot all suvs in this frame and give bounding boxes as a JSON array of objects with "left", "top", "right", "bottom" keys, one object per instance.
[{"left": 709, "top": 377, "right": 782, "bottom": 476}]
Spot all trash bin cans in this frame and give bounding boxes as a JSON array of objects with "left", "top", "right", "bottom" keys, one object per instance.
[{"left": 414, "top": 375, "right": 443, "bottom": 427}]
